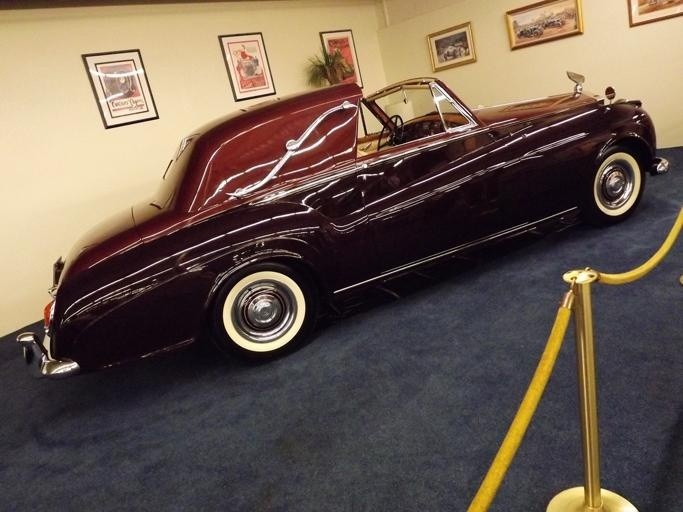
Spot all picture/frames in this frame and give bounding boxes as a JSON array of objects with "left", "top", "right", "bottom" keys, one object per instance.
[
  {"left": 505, "top": 0, "right": 584, "bottom": 51},
  {"left": 81, "top": 48, "right": 160, "bottom": 130},
  {"left": 425, "top": 20, "right": 477, "bottom": 73},
  {"left": 320, "top": 29, "right": 364, "bottom": 90},
  {"left": 217, "top": 32, "right": 276, "bottom": 103},
  {"left": 627, "top": 0, "right": 683, "bottom": 27}
]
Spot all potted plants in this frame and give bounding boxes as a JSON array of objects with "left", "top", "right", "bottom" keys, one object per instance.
[{"left": 305, "top": 44, "right": 355, "bottom": 88}]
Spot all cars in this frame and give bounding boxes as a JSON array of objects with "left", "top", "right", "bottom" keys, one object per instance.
[{"left": 16, "top": 70, "right": 669, "bottom": 379}]
